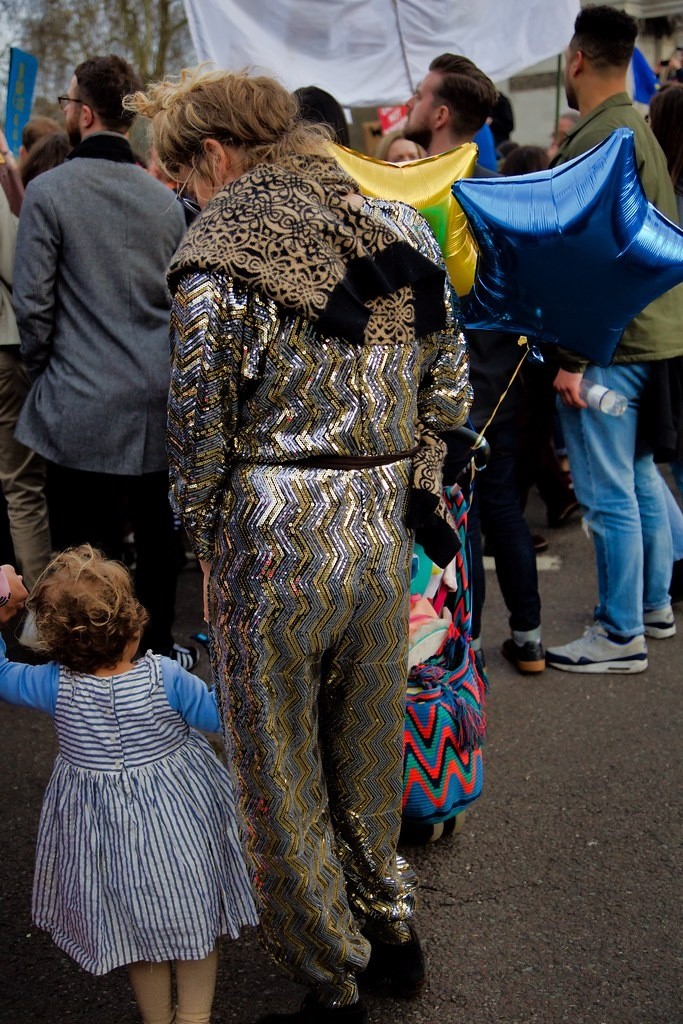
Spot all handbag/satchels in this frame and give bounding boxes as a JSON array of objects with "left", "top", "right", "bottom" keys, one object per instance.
[{"left": 398, "top": 636, "right": 487, "bottom": 824}]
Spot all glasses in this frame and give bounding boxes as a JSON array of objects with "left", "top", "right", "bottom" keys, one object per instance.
[
  {"left": 175, "top": 157, "right": 201, "bottom": 216},
  {"left": 58, "top": 94, "right": 84, "bottom": 104}
]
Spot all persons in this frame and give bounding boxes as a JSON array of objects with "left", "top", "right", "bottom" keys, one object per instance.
[
  {"left": 1, "top": 47, "right": 683, "bottom": 1024},
  {"left": 542, "top": 3, "right": 683, "bottom": 676}
]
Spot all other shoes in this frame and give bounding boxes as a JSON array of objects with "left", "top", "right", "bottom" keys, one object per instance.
[
  {"left": 532, "top": 534, "right": 550, "bottom": 550},
  {"left": 258, "top": 989, "right": 369, "bottom": 1024},
  {"left": 357, "top": 920, "right": 425, "bottom": 998},
  {"left": 171, "top": 644, "right": 200, "bottom": 672},
  {"left": 502, "top": 636, "right": 546, "bottom": 674},
  {"left": 414, "top": 810, "right": 466, "bottom": 842},
  {"left": 548, "top": 491, "right": 587, "bottom": 531}
]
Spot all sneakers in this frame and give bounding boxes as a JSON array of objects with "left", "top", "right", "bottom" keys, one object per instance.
[
  {"left": 545, "top": 621, "right": 650, "bottom": 674},
  {"left": 643, "top": 605, "right": 677, "bottom": 639}
]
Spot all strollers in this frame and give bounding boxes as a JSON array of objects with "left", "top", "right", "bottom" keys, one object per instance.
[{"left": 390, "top": 426, "right": 491, "bottom": 842}]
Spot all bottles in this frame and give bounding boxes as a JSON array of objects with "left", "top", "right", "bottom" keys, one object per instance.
[{"left": 579, "top": 378, "right": 628, "bottom": 417}]
